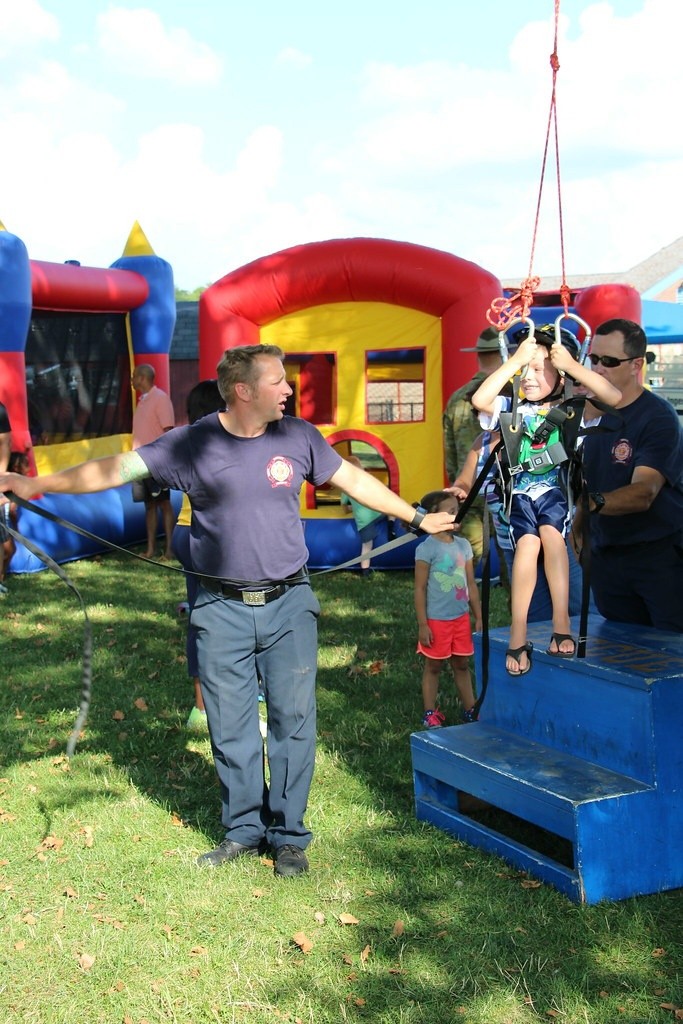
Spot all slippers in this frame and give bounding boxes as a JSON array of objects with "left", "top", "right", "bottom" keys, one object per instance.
[
  {"left": 506, "top": 641, "right": 533, "bottom": 677},
  {"left": 546, "top": 633, "right": 576, "bottom": 658}
]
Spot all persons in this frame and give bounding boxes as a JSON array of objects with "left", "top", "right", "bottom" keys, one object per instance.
[
  {"left": 340, "top": 456, "right": 397, "bottom": 577},
  {"left": 402, "top": 491, "right": 482, "bottom": 730},
  {"left": 131, "top": 364, "right": 174, "bottom": 562},
  {"left": 171, "top": 379, "right": 267, "bottom": 738},
  {"left": 442, "top": 326, "right": 600, "bottom": 623},
  {"left": 0, "top": 402, "right": 29, "bottom": 593},
  {"left": 572, "top": 319, "right": 683, "bottom": 634},
  {"left": 0, "top": 345, "right": 460, "bottom": 880},
  {"left": 471, "top": 323, "right": 623, "bottom": 676}
]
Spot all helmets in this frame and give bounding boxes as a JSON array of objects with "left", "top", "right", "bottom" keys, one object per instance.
[{"left": 512, "top": 324, "right": 582, "bottom": 363}]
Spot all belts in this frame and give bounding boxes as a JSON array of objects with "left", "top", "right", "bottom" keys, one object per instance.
[{"left": 200, "top": 564, "right": 308, "bottom": 606}]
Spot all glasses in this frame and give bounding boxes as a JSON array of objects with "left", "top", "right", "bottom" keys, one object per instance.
[{"left": 588, "top": 353, "right": 639, "bottom": 368}]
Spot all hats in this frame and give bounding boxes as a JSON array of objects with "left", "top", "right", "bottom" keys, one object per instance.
[{"left": 459, "top": 326, "right": 518, "bottom": 352}]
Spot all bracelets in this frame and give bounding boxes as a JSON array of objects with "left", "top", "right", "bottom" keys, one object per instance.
[{"left": 409, "top": 506, "right": 428, "bottom": 530}]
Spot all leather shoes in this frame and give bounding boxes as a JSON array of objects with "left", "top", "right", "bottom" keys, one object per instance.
[
  {"left": 198, "top": 839, "right": 266, "bottom": 866},
  {"left": 274, "top": 844, "right": 309, "bottom": 879}
]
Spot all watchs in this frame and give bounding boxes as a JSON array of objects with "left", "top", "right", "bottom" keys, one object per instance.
[{"left": 589, "top": 491, "right": 606, "bottom": 514}]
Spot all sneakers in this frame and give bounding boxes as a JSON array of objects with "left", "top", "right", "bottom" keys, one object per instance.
[
  {"left": 422, "top": 705, "right": 445, "bottom": 729},
  {"left": 462, "top": 708, "right": 479, "bottom": 724}
]
